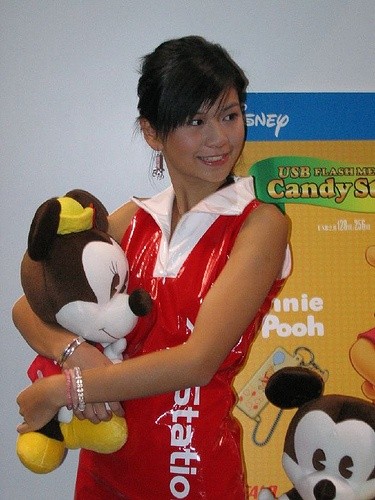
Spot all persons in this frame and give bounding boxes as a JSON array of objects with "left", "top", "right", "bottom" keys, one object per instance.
[{"left": 12, "top": 36, "right": 293, "bottom": 500}]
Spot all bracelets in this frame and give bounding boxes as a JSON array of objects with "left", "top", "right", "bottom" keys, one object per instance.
[
  {"left": 64, "top": 368, "right": 85, "bottom": 411},
  {"left": 58, "top": 336, "right": 88, "bottom": 366}
]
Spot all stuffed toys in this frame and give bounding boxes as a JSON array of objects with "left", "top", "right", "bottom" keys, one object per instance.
[
  {"left": 258, "top": 366, "right": 375, "bottom": 500},
  {"left": 12, "top": 188, "right": 151, "bottom": 475}
]
